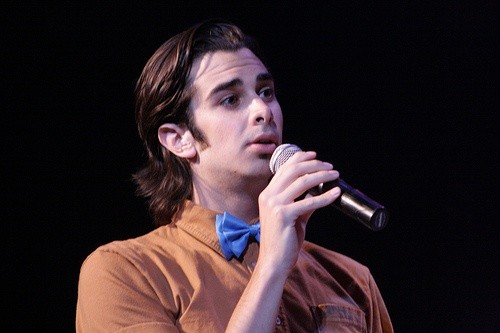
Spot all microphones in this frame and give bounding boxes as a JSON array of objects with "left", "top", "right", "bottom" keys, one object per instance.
[{"left": 269, "top": 144, "right": 387, "bottom": 230}]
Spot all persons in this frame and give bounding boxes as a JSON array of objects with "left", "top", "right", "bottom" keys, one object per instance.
[{"left": 75, "top": 22, "right": 397, "bottom": 333}]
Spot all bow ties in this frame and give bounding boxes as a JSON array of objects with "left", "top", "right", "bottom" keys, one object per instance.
[{"left": 216, "top": 213, "right": 262, "bottom": 262}]
